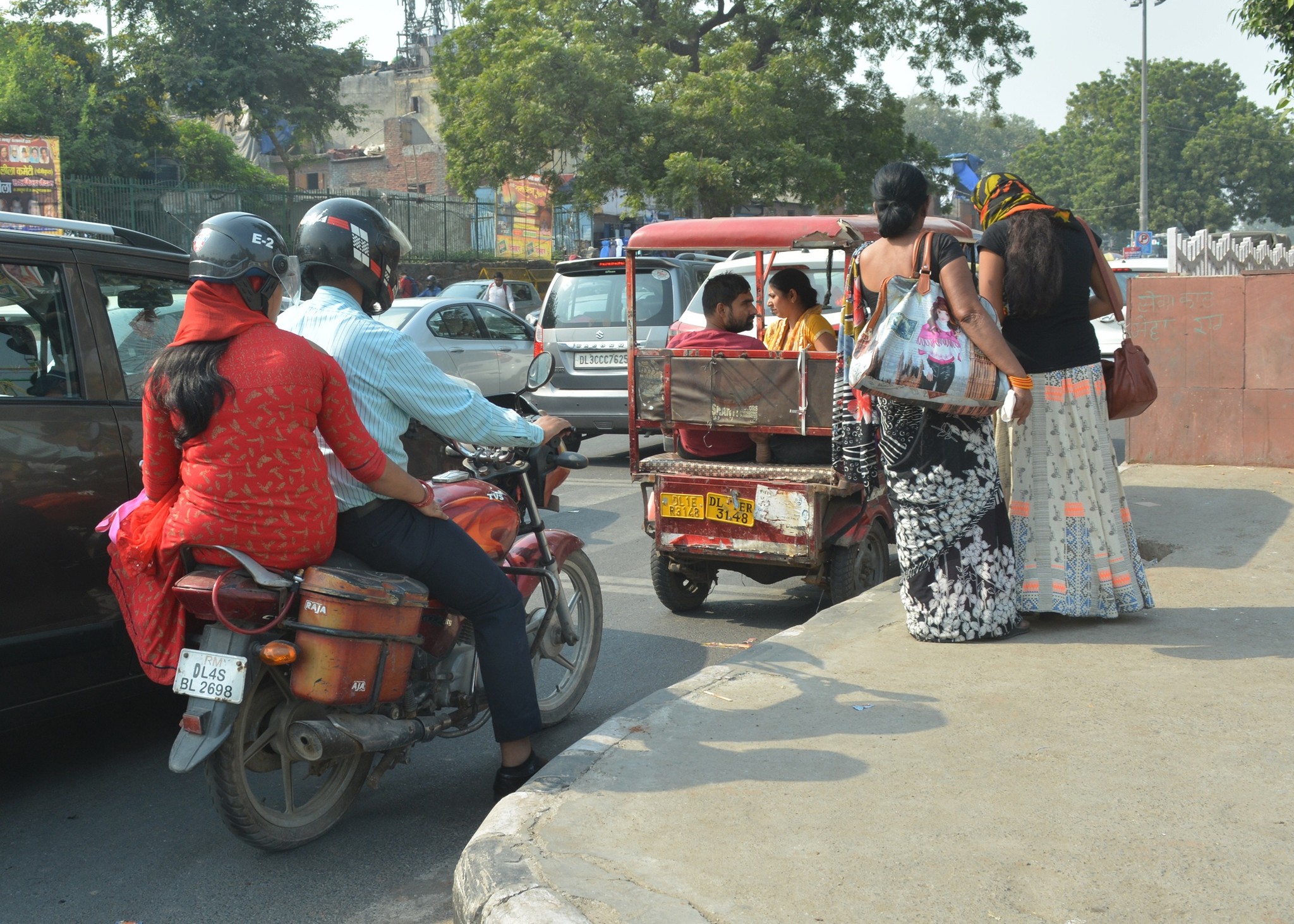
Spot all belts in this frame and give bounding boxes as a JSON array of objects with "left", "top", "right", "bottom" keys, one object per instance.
[{"left": 335, "top": 497, "right": 386, "bottom": 528}]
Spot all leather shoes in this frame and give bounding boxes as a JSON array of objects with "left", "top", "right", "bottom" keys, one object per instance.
[{"left": 493, "top": 755, "right": 550, "bottom": 802}]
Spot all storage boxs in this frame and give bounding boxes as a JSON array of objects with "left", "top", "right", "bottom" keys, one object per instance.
[{"left": 286, "top": 570, "right": 428, "bottom": 702}]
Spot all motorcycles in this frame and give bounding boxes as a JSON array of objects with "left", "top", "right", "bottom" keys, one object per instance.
[
  {"left": 166, "top": 352, "right": 603, "bottom": 856},
  {"left": 629, "top": 216, "right": 978, "bottom": 615}
]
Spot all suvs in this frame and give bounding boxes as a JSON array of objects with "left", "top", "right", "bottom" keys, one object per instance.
[
  {"left": 0, "top": 209, "right": 465, "bottom": 770},
  {"left": 666, "top": 249, "right": 847, "bottom": 346}
]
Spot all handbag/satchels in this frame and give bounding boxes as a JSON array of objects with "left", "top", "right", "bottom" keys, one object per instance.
[
  {"left": 1099, "top": 331, "right": 1160, "bottom": 421},
  {"left": 853, "top": 228, "right": 1013, "bottom": 419}
]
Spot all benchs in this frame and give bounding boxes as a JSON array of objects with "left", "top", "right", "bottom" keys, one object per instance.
[{"left": 633, "top": 346, "right": 862, "bottom": 494}]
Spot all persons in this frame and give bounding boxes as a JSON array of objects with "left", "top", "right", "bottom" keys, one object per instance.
[
  {"left": 30, "top": 148, "right": 39, "bottom": 163},
  {"left": 417, "top": 275, "right": 441, "bottom": 296},
  {"left": 26, "top": 291, "right": 109, "bottom": 396},
  {"left": 397, "top": 272, "right": 422, "bottom": 297},
  {"left": 483, "top": 272, "right": 515, "bottom": 329},
  {"left": 0, "top": 146, "right": 9, "bottom": 161},
  {"left": 832, "top": 163, "right": 1033, "bottom": 643},
  {"left": 275, "top": 197, "right": 573, "bottom": 802},
  {"left": 40, "top": 148, "right": 49, "bottom": 163},
  {"left": 748, "top": 267, "right": 837, "bottom": 462},
  {"left": 517, "top": 290, "right": 527, "bottom": 300},
  {"left": 107, "top": 211, "right": 449, "bottom": 686},
  {"left": 917, "top": 296, "right": 961, "bottom": 393},
  {"left": 666, "top": 273, "right": 806, "bottom": 463},
  {"left": 10, "top": 147, "right": 19, "bottom": 161},
  {"left": 0, "top": 197, "right": 55, "bottom": 225},
  {"left": 970, "top": 172, "right": 1154, "bottom": 619},
  {"left": 20, "top": 148, "right": 29, "bottom": 163}
]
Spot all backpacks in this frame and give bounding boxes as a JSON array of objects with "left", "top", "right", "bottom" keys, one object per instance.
[{"left": 404, "top": 276, "right": 419, "bottom": 297}]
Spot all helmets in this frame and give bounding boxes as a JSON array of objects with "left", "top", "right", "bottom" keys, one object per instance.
[
  {"left": 295, "top": 196, "right": 400, "bottom": 316},
  {"left": 187, "top": 209, "right": 296, "bottom": 312},
  {"left": 426, "top": 275, "right": 438, "bottom": 289}
]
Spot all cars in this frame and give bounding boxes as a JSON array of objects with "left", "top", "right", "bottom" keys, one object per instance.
[
  {"left": 1090, "top": 258, "right": 1171, "bottom": 360},
  {"left": 525, "top": 281, "right": 656, "bottom": 326},
  {"left": 371, "top": 296, "right": 536, "bottom": 397},
  {"left": 0, "top": 290, "right": 304, "bottom": 398}
]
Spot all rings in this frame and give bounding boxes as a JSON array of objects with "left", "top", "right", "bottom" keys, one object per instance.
[{"left": 435, "top": 504, "right": 439, "bottom": 509}]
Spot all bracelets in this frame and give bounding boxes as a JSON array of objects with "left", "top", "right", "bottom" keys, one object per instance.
[
  {"left": 1008, "top": 374, "right": 1033, "bottom": 389},
  {"left": 407, "top": 479, "right": 434, "bottom": 507}
]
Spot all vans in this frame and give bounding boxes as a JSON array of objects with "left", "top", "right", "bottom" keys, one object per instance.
[
  {"left": 434, "top": 279, "right": 527, "bottom": 324},
  {"left": 523, "top": 253, "right": 732, "bottom": 453}
]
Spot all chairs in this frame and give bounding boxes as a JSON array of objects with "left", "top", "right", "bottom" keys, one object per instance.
[
  {"left": 0, "top": 323, "right": 41, "bottom": 399},
  {"left": 440, "top": 311, "right": 473, "bottom": 340}
]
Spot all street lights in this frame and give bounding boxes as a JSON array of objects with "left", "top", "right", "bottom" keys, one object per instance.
[{"left": 1130, "top": 0, "right": 1166, "bottom": 232}]
